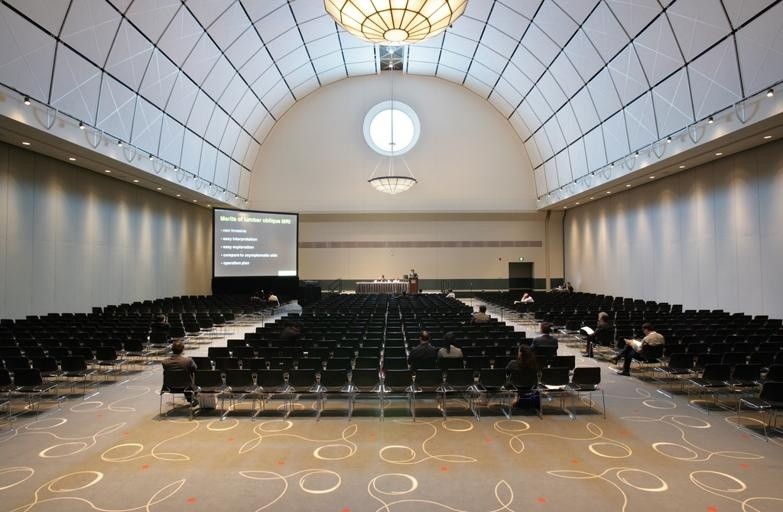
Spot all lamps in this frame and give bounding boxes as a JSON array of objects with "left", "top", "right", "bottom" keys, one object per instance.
[
  {"left": 367, "top": 44, "right": 417, "bottom": 197},
  {"left": 318, "top": 0, "right": 468, "bottom": 50}
]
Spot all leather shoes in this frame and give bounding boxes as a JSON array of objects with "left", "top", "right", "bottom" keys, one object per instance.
[
  {"left": 617, "top": 371, "right": 630, "bottom": 376},
  {"left": 606, "top": 358, "right": 617, "bottom": 365}
]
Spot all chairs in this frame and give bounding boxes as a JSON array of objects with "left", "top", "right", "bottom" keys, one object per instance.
[
  {"left": 0, "top": 297, "right": 155, "bottom": 429},
  {"left": 158, "top": 283, "right": 612, "bottom": 421},
  {"left": 613, "top": 298, "right": 782, "bottom": 441}
]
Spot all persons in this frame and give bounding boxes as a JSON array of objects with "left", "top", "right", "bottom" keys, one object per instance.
[
  {"left": 579, "top": 311, "right": 611, "bottom": 358},
  {"left": 558, "top": 282, "right": 567, "bottom": 290},
  {"left": 469, "top": 305, "right": 489, "bottom": 324},
  {"left": 160, "top": 340, "right": 199, "bottom": 403},
  {"left": 407, "top": 330, "right": 437, "bottom": 370},
  {"left": 513, "top": 292, "right": 534, "bottom": 318},
  {"left": 436, "top": 332, "right": 464, "bottom": 366},
  {"left": 504, "top": 344, "right": 539, "bottom": 405},
  {"left": 266, "top": 291, "right": 280, "bottom": 316},
  {"left": 376, "top": 274, "right": 387, "bottom": 282},
  {"left": 249, "top": 289, "right": 266, "bottom": 303},
  {"left": 408, "top": 268, "right": 419, "bottom": 294},
  {"left": 529, "top": 322, "right": 559, "bottom": 354},
  {"left": 564, "top": 280, "right": 573, "bottom": 293},
  {"left": 445, "top": 288, "right": 455, "bottom": 299},
  {"left": 605, "top": 323, "right": 666, "bottom": 377}
]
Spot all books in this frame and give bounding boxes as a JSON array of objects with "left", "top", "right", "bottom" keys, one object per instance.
[
  {"left": 623, "top": 337, "right": 641, "bottom": 348},
  {"left": 580, "top": 326, "right": 594, "bottom": 336}
]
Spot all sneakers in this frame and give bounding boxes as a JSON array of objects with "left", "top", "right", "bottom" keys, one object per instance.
[{"left": 580, "top": 351, "right": 593, "bottom": 358}]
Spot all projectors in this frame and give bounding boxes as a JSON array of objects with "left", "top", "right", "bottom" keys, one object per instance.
[{"left": 304, "top": 281, "right": 319, "bottom": 285}]
